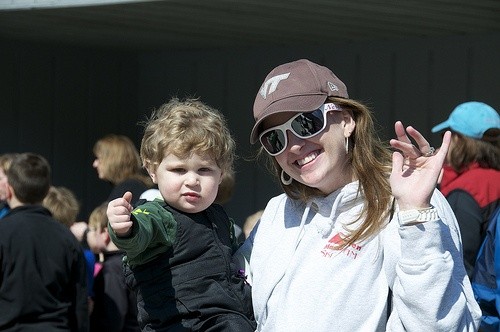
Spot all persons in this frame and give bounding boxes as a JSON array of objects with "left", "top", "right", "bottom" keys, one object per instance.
[
  {"left": 232, "top": 58, "right": 485, "bottom": 332},
  {"left": 0, "top": 152, "right": 90, "bottom": 332},
  {"left": 432, "top": 101, "right": 500, "bottom": 284},
  {"left": 105, "top": 92, "right": 258, "bottom": 332},
  {"left": 0, "top": 129, "right": 268, "bottom": 332},
  {"left": 464, "top": 204, "right": 500, "bottom": 332}
]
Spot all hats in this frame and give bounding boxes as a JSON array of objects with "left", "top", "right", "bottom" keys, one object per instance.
[
  {"left": 249, "top": 59, "right": 348, "bottom": 144},
  {"left": 432, "top": 101, "right": 500, "bottom": 141}
]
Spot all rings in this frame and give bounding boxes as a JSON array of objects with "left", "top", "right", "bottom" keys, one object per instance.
[{"left": 421, "top": 147, "right": 436, "bottom": 157}]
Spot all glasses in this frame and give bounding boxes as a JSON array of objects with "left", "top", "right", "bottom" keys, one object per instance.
[{"left": 258, "top": 102, "right": 344, "bottom": 156}]
